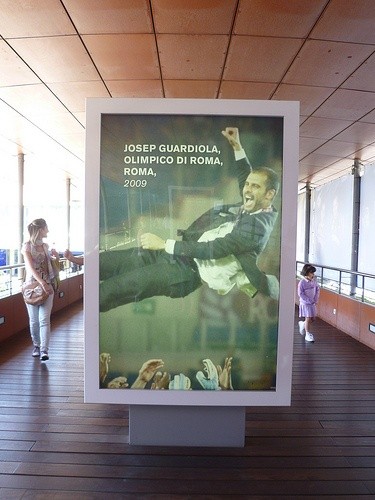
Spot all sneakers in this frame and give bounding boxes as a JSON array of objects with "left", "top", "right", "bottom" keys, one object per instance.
[
  {"left": 305, "top": 334, "right": 315, "bottom": 341},
  {"left": 40, "top": 351, "right": 49, "bottom": 361},
  {"left": 298, "top": 321, "right": 305, "bottom": 335},
  {"left": 32, "top": 346, "right": 40, "bottom": 357}
]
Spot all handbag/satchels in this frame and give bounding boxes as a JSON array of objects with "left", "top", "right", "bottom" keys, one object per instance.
[{"left": 22, "top": 280, "right": 49, "bottom": 305}]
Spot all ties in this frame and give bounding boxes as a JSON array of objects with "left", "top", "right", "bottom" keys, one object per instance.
[{"left": 177, "top": 208, "right": 243, "bottom": 236}]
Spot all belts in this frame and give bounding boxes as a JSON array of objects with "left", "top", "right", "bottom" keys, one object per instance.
[{"left": 188, "top": 255, "right": 199, "bottom": 272}]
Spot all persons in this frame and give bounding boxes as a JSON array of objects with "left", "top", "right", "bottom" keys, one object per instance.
[
  {"left": 99, "top": 127, "right": 280, "bottom": 313},
  {"left": 64, "top": 249, "right": 83, "bottom": 265},
  {"left": 298, "top": 264, "right": 319, "bottom": 341},
  {"left": 21, "top": 218, "right": 60, "bottom": 361},
  {"left": 99, "top": 353, "right": 234, "bottom": 391}
]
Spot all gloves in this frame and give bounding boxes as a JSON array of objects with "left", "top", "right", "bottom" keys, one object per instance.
[
  {"left": 195, "top": 359, "right": 219, "bottom": 390},
  {"left": 168, "top": 373, "right": 192, "bottom": 390}
]
